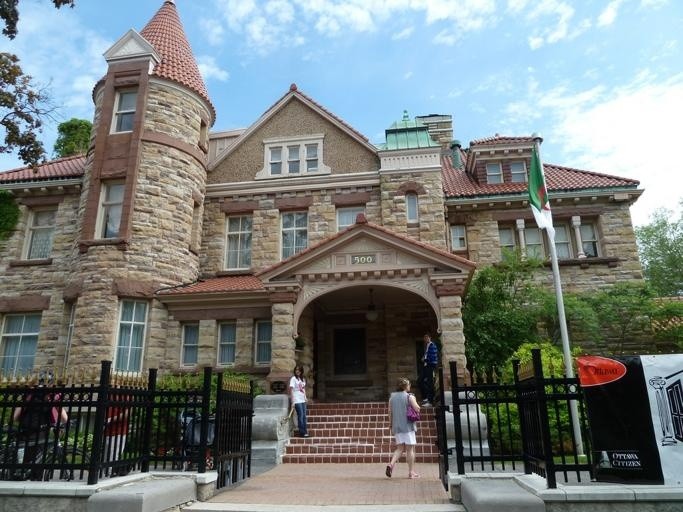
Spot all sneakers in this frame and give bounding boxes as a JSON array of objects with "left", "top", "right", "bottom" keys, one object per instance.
[
  {"left": 386, "top": 464, "right": 393, "bottom": 477},
  {"left": 409, "top": 472, "right": 420, "bottom": 479},
  {"left": 422, "top": 398, "right": 433, "bottom": 407}
]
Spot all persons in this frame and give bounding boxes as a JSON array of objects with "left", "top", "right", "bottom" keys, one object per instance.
[
  {"left": 100, "top": 373, "right": 135, "bottom": 476},
  {"left": 386, "top": 377, "right": 421, "bottom": 479},
  {"left": 14, "top": 370, "right": 68, "bottom": 465},
  {"left": 420, "top": 333, "right": 438, "bottom": 407},
  {"left": 289, "top": 364, "right": 309, "bottom": 437}
]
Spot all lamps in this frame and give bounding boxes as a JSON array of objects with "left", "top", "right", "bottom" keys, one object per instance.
[{"left": 365, "top": 288, "right": 380, "bottom": 322}]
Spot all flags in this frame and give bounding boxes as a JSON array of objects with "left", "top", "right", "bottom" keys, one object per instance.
[{"left": 527, "top": 141, "right": 557, "bottom": 246}]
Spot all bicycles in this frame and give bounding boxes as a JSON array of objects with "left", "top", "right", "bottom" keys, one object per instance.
[{"left": 0, "top": 420, "right": 91, "bottom": 481}]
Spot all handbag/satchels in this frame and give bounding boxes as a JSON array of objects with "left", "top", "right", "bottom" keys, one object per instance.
[{"left": 406, "top": 405, "right": 420, "bottom": 421}]
